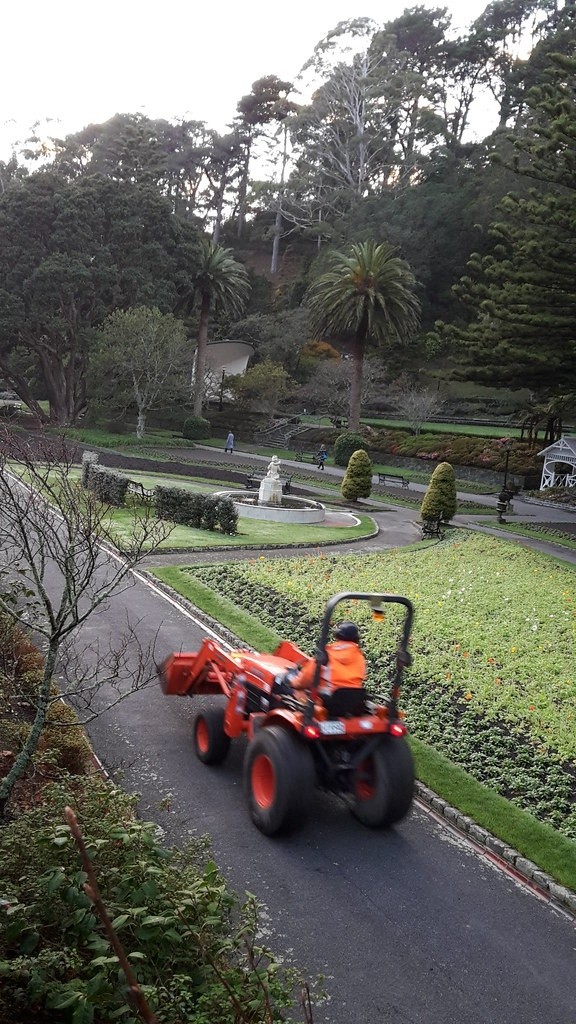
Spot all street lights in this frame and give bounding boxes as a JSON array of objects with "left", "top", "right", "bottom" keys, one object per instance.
[
  {"left": 218, "top": 365, "right": 227, "bottom": 411},
  {"left": 501, "top": 437, "right": 513, "bottom": 490}
]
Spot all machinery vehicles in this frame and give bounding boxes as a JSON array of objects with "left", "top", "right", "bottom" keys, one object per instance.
[{"left": 156, "top": 591, "right": 415, "bottom": 840}]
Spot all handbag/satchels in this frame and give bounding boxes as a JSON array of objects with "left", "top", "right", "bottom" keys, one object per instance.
[{"left": 323, "top": 454, "right": 328, "bottom": 459}]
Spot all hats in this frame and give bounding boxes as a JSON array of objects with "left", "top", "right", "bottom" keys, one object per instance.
[{"left": 337, "top": 623, "right": 357, "bottom": 640}]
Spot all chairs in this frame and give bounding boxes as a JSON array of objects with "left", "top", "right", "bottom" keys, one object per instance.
[{"left": 318, "top": 687, "right": 367, "bottom": 719}]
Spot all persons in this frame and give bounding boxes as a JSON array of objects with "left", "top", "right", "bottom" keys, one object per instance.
[
  {"left": 283, "top": 623, "right": 367, "bottom": 719},
  {"left": 267, "top": 455, "right": 281, "bottom": 478},
  {"left": 315, "top": 444, "right": 327, "bottom": 471},
  {"left": 225, "top": 430, "right": 234, "bottom": 454}
]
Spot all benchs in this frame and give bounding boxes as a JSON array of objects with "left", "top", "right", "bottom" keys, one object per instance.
[
  {"left": 126, "top": 480, "right": 156, "bottom": 506},
  {"left": 378, "top": 473, "right": 409, "bottom": 489},
  {"left": 421, "top": 517, "right": 444, "bottom": 540},
  {"left": 297, "top": 450, "right": 321, "bottom": 463},
  {"left": 244, "top": 469, "right": 294, "bottom": 495}
]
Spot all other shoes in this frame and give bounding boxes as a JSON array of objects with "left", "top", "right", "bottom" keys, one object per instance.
[
  {"left": 321, "top": 469, "right": 324, "bottom": 470},
  {"left": 317, "top": 467, "right": 319, "bottom": 468}
]
